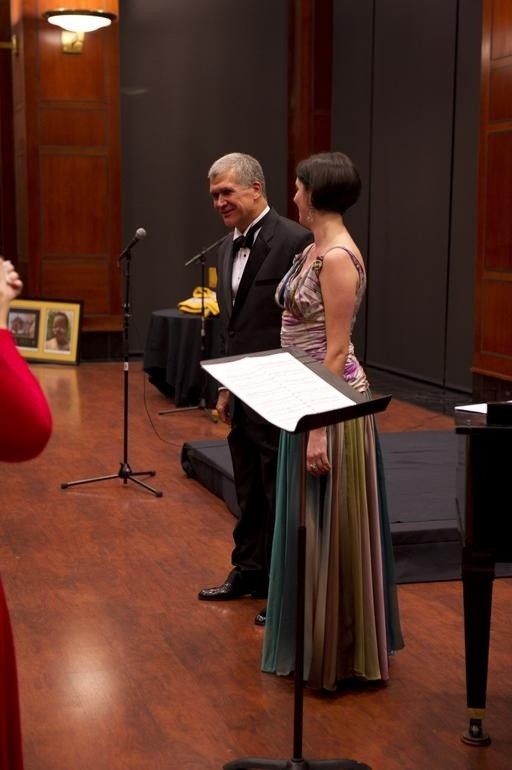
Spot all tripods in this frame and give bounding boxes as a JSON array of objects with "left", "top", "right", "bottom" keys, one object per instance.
[
  {"left": 157, "top": 255, "right": 219, "bottom": 424},
  {"left": 61, "top": 262, "right": 163, "bottom": 497}
]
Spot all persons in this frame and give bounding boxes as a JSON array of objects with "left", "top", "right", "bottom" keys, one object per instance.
[
  {"left": 262, "top": 152, "right": 405, "bottom": 694},
  {"left": 46, "top": 313, "right": 70, "bottom": 352},
  {"left": 197, "top": 152, "right": 313, "bottom": 600},
  {"left": 0, "top": 255, "right": 53, "bottom": 768}
]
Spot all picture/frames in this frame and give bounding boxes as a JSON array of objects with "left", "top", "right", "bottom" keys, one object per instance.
[{"left": 6, "top": 294, "right": 87, "bottom": 367}]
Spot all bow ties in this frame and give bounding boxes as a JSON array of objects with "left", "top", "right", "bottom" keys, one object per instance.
[{"left": 234, "top": 235, "right": 253, "bottom": 252}]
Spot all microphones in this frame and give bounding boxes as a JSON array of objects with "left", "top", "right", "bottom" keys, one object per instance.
[{"left": 118, "top": 228, "right": 146, "bottom": 261}]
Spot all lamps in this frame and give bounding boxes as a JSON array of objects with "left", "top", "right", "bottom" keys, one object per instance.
[{"left": 38, "top": 0, "right": 122, "bottom": 55}]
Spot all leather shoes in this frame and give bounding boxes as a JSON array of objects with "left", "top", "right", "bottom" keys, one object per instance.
[{"left": 200, "top": 568, "right": 268, "bottom": 599}]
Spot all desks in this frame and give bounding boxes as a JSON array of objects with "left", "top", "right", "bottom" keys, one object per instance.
[
  {"left": 448, "top": 399, "right": 511, "bottom": 748},
  {"left": 147, "top": 308, "right": 226, "bottom": 412}
]
